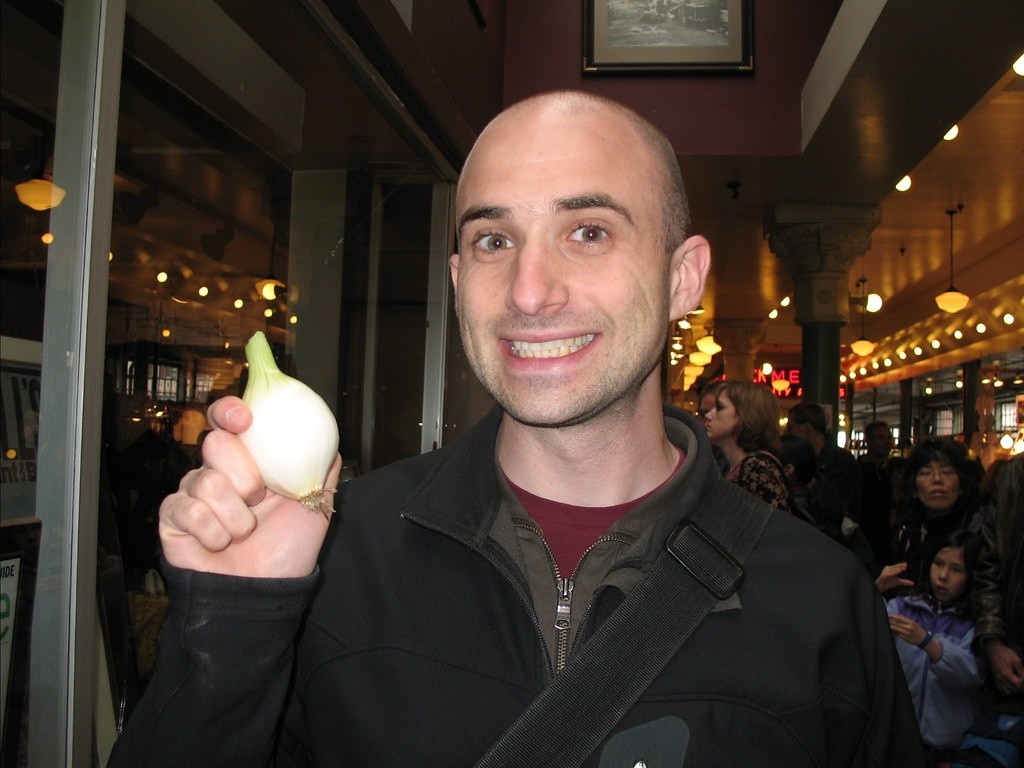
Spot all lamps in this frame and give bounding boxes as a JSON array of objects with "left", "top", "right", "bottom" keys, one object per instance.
[
  {"left": 675, "top": 320, "right": 727, "bottom": 392},
  {"left": 118, "top": 184, "right": 151, "bottom": 225},
  {"left": 771, "top": 345, "right": 789, "bottom": 391},
  {"left": 934, "top": 198, "right": 971, "bottom": 315},
  {"left": 848, "top": 272, "right": 883, "bottom": 357},
  {"left": 254, "top": 202, "right": 287, "bottom": 300},
  {"left": 15, "top": 123, "right": 54, "bottom": 214},
  {"left": 200, "top": 221, "right": 234, "bottom": 262}
]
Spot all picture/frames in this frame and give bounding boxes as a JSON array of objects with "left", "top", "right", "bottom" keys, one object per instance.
[{"left": 582, "top": 1, "right": 755, "bottom": 78}]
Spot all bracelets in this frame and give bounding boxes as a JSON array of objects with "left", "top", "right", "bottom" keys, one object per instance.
[{"left": 918, "top": 632, "right": 933, "bottom": 649}]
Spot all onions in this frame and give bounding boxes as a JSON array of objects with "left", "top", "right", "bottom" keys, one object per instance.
[{"left": 235, "top": 332, "right": 339, "bottom": 511}]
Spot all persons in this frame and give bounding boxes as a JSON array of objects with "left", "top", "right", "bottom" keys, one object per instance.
[
  {"left": 698, "top": 381, "right": 1024, "bottom": 768},
  {"left": 106, "top": 92, "right": 928, "bottom": 768}
]
